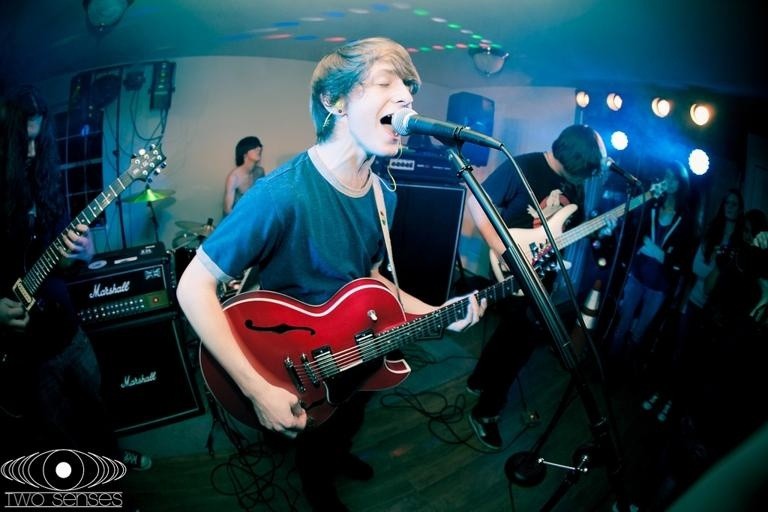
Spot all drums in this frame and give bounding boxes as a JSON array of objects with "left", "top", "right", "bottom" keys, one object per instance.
[{"left": 175, "top": 235, "right": 203, "bottom": 289}]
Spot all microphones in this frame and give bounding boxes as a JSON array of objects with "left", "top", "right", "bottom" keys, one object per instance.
[
  {"left": 392, "top": 107, "right": 503, "bottom": 152},
  {"left": 605, "top": 158, "right": 642, "bottom": 189}
]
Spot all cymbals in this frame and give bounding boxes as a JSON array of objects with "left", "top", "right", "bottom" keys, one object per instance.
[
  {"left": 123, "top": 190, "right": 177, "bottom": 203},
  {"left": 175, "top": 221, "right": 217, "bottom": 238}
]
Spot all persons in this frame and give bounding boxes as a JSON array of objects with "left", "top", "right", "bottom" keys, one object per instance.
[
  {"left": 0, "top": 86, "right": 153, "bottom": 478},
  {"left": 613, "top": 160, "right": 694, "bottom": 352},
  {"left": 456, "top": 123, "right": 611, "bottom": 454},
  {"left": 221, "top": 135, "right": 265, "bottom": 218},
  {"left": 702, "top": 206, "right": 767, "bottom": 370},
  {"left": 171, "top": 33, "right": 489, "bottom": 512},
  {"left": 640, "top": 187, "right": 749, "bottom": 424}
]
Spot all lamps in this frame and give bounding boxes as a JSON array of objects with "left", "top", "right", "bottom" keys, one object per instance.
[
  {"left": 69, "top": 61, "right": 175, "bottom": 248},
  {"left": 574, "top": 82, "right": 747, "bottom": 180},
  {"left": 468, "top": 48, "right": 510, "bottom": 75},
  {"left": 83, "top": 0, "right": 134, "bottom": 33}
]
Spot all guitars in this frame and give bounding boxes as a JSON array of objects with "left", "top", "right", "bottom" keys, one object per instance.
[
  {"left": 490, "top": 181, "right": 666, "bottom": 297},
  {"left": 1, "top": 142, "right": 168, "bottom": 418},
  {"left": 197, "top": 239, "right": 560, "bottom": 430}
]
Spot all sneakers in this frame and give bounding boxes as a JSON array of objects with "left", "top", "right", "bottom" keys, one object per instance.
[
  {"left": 468, "top": 374, "right": 488, "bottom": 394},
  {"left": 471, "top": 407, "right": 504, "bottom": 451},
  {"left": 113, "top": 447, "right": 153, "bottom": 473},
  {"left": 305, "top": 453, "right": 372, "bottom": 511}
]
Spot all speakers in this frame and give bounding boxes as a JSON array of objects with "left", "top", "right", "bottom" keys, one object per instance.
[
  {"left": 446, "top": 92, "right": 498, "bottom": 168},
  {"left": 87, "top": 311, "right": 205, "bottom": 437}
]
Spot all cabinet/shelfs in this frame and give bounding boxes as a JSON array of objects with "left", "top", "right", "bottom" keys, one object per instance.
[{"left": 50, "top": 104, "right": 106, "bottom": 230}]
